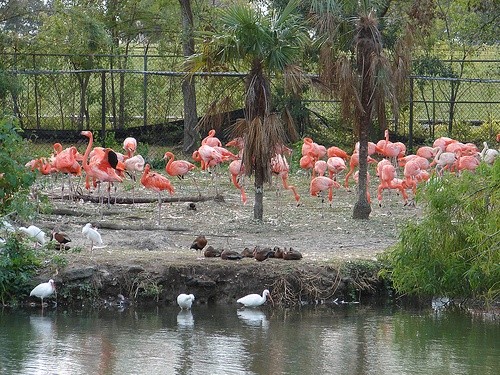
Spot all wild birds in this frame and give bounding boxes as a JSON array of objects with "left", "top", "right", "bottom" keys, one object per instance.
[
  {"left": 190, "top": 234, "right": 208, "bottom": 251},
  {"left": 203, "top": 245, "right": 302, "bottom": 261},
  {"left": 55, "top": 232, "right": 71, "bottom": 253},
  {"left": 83, "top": 222, "right": 104, "bottom": 256},
  {"left": 177, "top": 292, "right": 195, "bottom": 311},
  {"left": 29, "top": 129, "right": 500, "bottom": 217},
  {"left": 141, "top": 162, "right": 176, "bottom": 226},
  {"left": 236, "top": 290, "right": 275, "bottom": 307},
  {"left": 19, "top": 226, "right": 46, "bottom": 248},
  {"left": 29, "top": 279, "right": 55, "bottom": 309}
]
